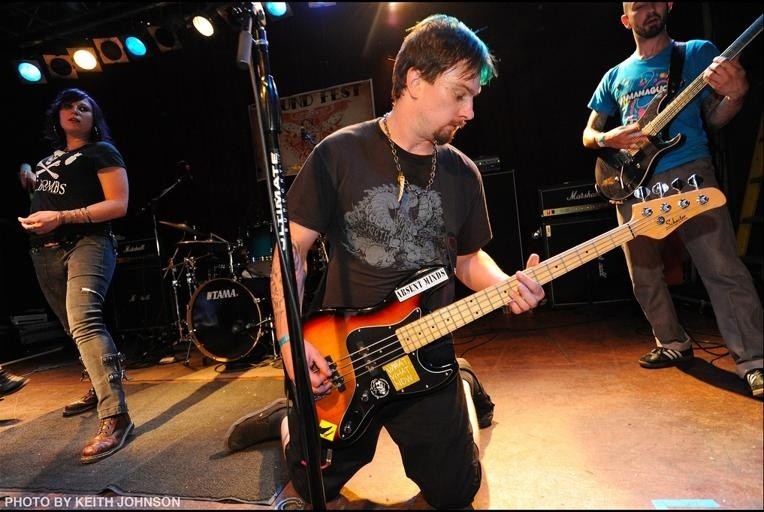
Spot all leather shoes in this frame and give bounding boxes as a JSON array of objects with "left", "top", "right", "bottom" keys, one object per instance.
[
  {"left": 2, "top": 368, "right": 30, "bottom": 396},
  {"left": 63, "top": 386, "right": 98, "bottom": 415},
  {"left": 79, "top": 412, "right": 135, "bottom": 464}
]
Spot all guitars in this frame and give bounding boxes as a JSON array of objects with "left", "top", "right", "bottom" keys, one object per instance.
[
  {"left": 593, "top": 14, "right": 763, "bottom": 204},
  {"left": 284, "top": 173, "right": 727, "bottom": 442}
]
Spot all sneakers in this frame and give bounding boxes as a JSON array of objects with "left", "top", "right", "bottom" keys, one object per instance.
[
  {"left": 638, "top": 347, "right": 695, "bottom": 368},
  {"left": 225, "top": 397, "right": 293, "bottom": 453},
  {"left": 747, "top": 369, "right": 763, "bottom": 397},
  {"left": 455, "top": 358, "right": 495, "bottom": 429}
]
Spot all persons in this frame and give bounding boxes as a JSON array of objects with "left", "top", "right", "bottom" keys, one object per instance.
[
  {"left": 580, "top": 2, "right": 763, "bottom": 397},
  {"left": 17, "top": 87, "right": 134, "bottom": 464},
  {"left": 222, "top": 13, "right": 549, "bottom": 510}
]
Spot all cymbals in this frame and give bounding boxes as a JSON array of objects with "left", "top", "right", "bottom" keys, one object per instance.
[
  {"left": 159, "top": 221, "right": 207, "bottom": 236},
  {"left": 177, "top": 239, "right": 232, "bottom": 245}
]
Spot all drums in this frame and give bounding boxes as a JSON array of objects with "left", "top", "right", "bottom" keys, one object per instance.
[
  {"left": 187, "top": 277, "right": 272, "bottom": 365},
  {"left": 245, "top": 218, "right": 275, "bottom": 275}
]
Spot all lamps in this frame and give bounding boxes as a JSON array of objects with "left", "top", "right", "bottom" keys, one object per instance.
[{"left": 14, "top": 0, "right": 293, "bottom": 87}]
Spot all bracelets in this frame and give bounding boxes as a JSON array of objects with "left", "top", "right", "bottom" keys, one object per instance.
[
  {"left": 725, "top": 94, "right": 740, "bottom": 101},
  {"left": 56, "top": 206, "right": 92, "bottom": 225},
  {"left": 277, "top": 332, "right": 290, "bottom": 347},
  {"left": 594, "top": 131, "right": 608, "bottom": 148}
]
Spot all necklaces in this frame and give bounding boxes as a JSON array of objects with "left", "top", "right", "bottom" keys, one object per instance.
[{"left": 380, "top": 110, "right": 439, "bottom": 203}]
